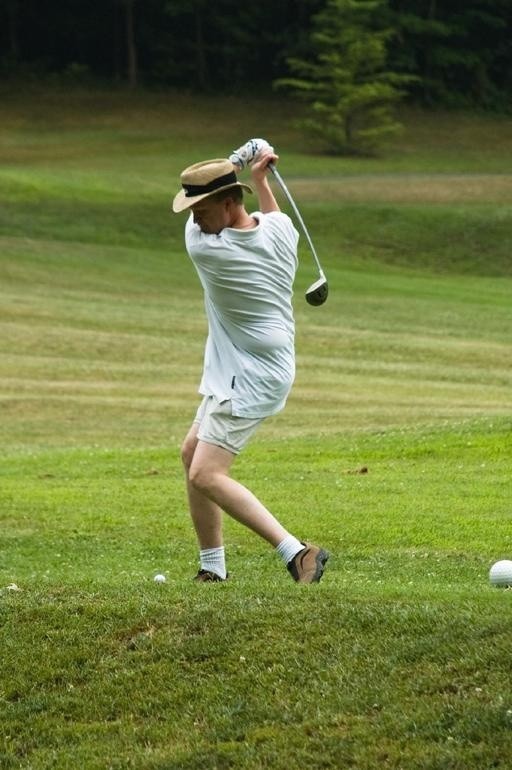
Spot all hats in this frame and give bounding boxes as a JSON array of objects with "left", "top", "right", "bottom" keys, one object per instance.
[{"left": 172, "top": 158, "right": 252, "bottom": 212}]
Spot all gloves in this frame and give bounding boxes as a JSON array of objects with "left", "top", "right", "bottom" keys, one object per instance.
[{"left": 229, "top": 138, "right": 273, "bottom": 170}]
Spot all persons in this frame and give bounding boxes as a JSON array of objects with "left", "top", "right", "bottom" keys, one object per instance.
[{"left": 171, "top": 138, "right": 329, "bottom": 586}]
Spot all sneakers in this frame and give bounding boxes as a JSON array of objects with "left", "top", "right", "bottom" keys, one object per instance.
[
  {"left": 190, "top": 568, "right": 230, "bottom": 583},
  {"left": 286, "top": 541, "right": 331, "bottom": 587}
]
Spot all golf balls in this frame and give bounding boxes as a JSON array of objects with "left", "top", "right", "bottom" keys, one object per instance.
[
  {"left": 155, "top": 575, "right": 165, "bottom": 582},
  {"left": 489, "top": 560, "right": 512, "bottom": 585}
]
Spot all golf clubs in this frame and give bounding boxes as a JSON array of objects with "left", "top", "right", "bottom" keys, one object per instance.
[{"left": 268, "top": 161, "right": 328, "bottom": 306}]
